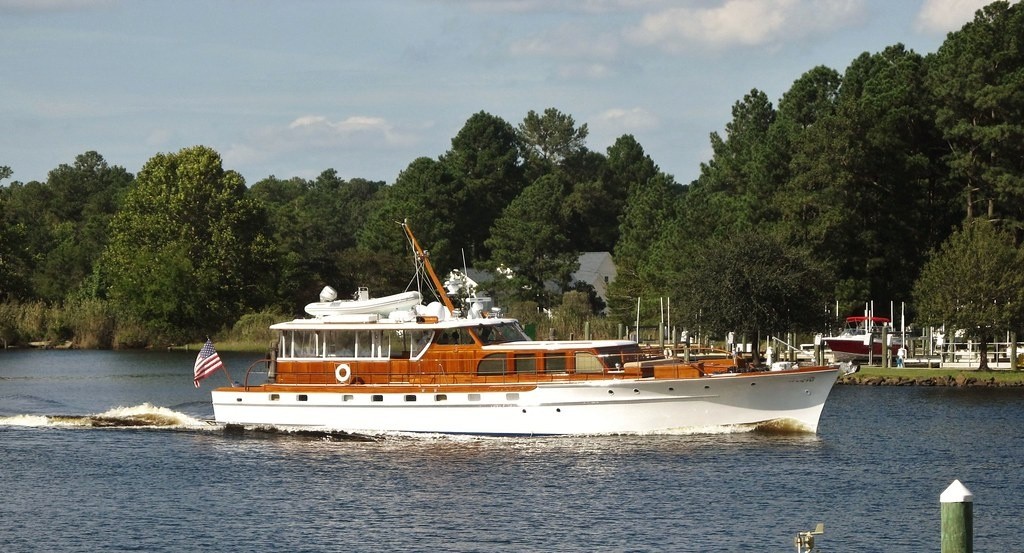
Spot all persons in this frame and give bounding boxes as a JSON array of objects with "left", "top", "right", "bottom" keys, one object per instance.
[{"left": 415, "top": 330, "right": 460, "bottom": 344}]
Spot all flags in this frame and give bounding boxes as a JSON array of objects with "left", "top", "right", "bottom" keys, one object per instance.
[{"left": 193, "top": 338, "right": 224, "bottom": 388}]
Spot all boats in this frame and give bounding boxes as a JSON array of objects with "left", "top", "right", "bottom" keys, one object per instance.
[
  {"left": 193, "top": 216, "right": 842, "bottom": 439},
  {"left": 820, "top": 316, "right": 909, "bottom": 363}
]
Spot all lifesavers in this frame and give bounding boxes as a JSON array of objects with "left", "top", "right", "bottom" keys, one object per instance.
[
  {"left": 663, "top": 348, "right": 672, "bottom": 359},
  {"left": 336, "top": 364, "right": 350, "bottom": 381}
]
